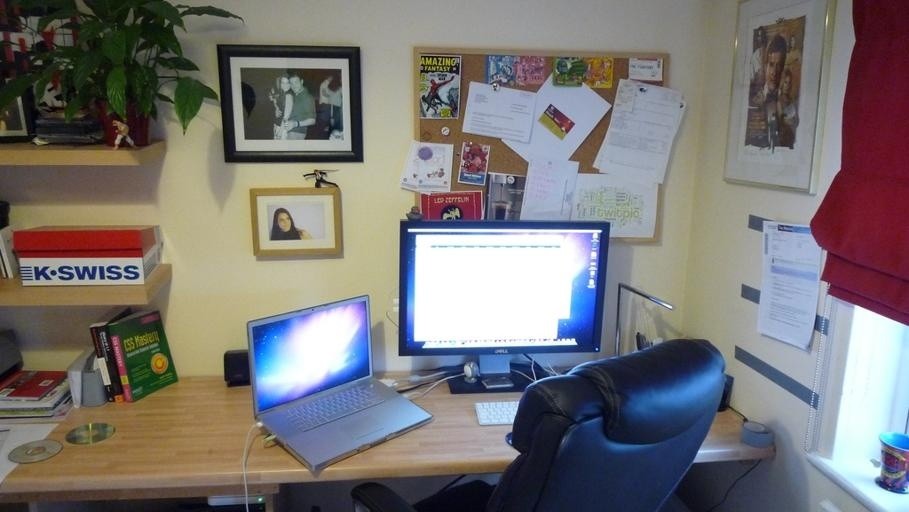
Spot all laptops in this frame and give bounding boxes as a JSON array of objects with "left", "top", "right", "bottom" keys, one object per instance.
[{"left": 245, "top": 293, "right": 434, "bottom": 473}]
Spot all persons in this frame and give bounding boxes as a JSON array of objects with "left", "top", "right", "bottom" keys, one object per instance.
[
  {"left": 750, "top": 27, "right": 799, "bottom": 148},
  {"left": 426, "top": 77, "right": 455, "bottom": 111},
  {"left": 113, "top": 120, "right": 139, "bottom": 149},
  {"left": 271, "top": 208, "right": 310, "bottom": 239},
  {"left": 270, "top": 73, "right": 343, "bottom": 140}
]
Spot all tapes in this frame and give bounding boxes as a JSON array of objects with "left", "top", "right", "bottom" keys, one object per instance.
[{"left": 740, "top": 421, "right": 774, "bottom": 448}]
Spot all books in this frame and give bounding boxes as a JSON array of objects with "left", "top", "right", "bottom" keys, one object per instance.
[{"left": 0, "top": 306, "right": 178, "bottom": 418}]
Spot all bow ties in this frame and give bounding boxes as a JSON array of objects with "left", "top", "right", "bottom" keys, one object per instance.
[{"left": 766, "top": 94, "right": 776, "bottom": 102}]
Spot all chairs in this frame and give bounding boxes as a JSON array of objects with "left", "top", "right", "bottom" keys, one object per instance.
[{"left": 361, "top": 327, "right": 731, "bottom": 509}]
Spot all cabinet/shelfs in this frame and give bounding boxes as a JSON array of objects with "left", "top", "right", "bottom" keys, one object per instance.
[{"left": 1, "top": 139, "right": 170, "bottom": 303}]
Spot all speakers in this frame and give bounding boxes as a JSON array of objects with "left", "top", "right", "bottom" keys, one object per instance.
[{"left": 222, "top": 349, "right": 250, "bottom": 386}]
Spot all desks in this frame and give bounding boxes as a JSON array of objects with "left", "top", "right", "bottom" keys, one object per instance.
[{"left": 3, "top": 375, "right": 779, "bottom": 511}]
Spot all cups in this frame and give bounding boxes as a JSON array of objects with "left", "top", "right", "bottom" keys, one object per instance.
[{"left": 879, "top": 432, "right": 909, "bottom": 490}]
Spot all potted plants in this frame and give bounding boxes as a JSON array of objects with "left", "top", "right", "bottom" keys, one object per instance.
[{"left": 0, "top": 0, "right": 245, "bottom": 142}]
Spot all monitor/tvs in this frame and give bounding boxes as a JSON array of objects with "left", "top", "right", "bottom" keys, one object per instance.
[{"left": 397, "top": 218, "right": 610, "bottom": 396}]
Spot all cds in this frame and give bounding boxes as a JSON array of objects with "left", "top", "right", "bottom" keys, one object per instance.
[
  {"left": 65, "top": 422, "right": 115, "bottom": 446},
  {"left": 7, "top": 439, "right": 62, "bottom": 464}
]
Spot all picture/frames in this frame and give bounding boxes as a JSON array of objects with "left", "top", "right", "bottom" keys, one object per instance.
[
  {"left": 215, "top": 40, "right": 365, "bottom": 262},
  {"left": 721, "top": 1, "right": 836, "bottom": 198}
]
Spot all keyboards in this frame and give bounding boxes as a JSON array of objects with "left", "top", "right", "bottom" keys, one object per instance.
[{"left": 473, "top": 402, "right": 518, "bottom": 428}]
